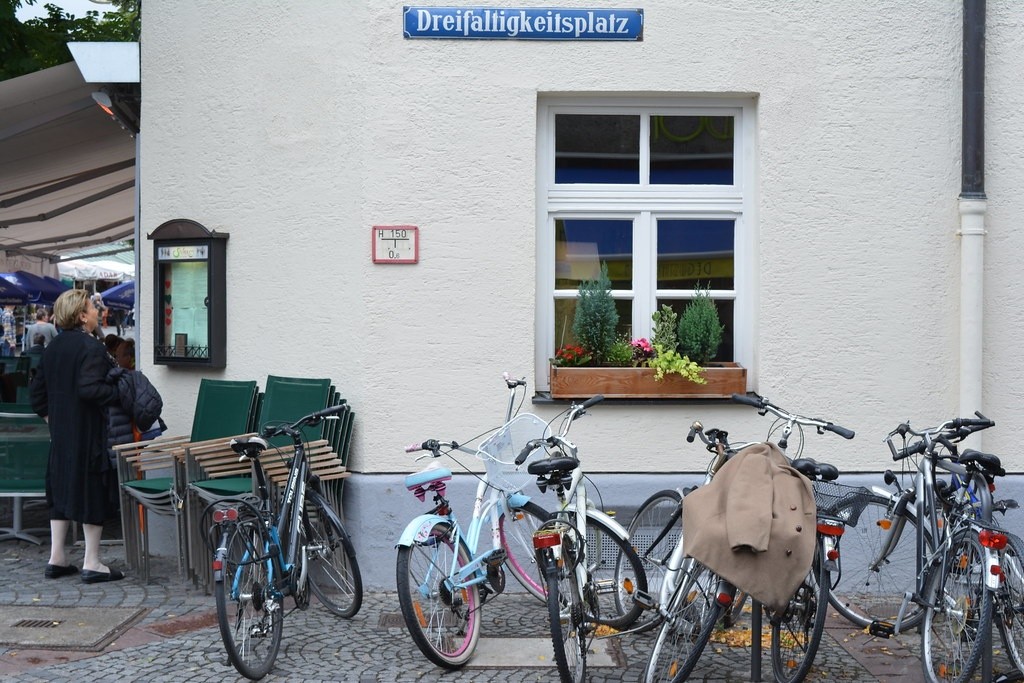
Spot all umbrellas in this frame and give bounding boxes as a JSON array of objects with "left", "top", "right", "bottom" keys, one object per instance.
[{"left": 0, "top": 270, "right": 136, "bottom": 355}]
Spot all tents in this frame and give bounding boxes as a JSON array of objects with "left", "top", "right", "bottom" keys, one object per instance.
[{"left": 58, "top": 256, "right": 135, "bottom": 293}]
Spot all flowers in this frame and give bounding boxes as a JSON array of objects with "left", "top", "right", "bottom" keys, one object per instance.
[{"left": 549, "top": 259, "right": 725, "bottom": 385}]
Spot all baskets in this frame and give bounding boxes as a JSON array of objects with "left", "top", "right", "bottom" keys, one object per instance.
[
  {"left": 813, "top": 477, "right": 875, "bottom": 528},
  {"left": 476, "top": 414, "right": 554, "bottom": 493}
]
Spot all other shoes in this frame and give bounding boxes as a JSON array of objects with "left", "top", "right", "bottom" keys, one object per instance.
[
  {"left": 45, "top": 563, "right": 78, "bottom": 578},
  {"left": 81, "top": 564, "right": 126, "bottom": 583}
]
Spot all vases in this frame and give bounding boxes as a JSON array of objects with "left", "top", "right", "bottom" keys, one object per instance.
[{"left": 550, "top": 363, "right": 747, "bottom": 398}]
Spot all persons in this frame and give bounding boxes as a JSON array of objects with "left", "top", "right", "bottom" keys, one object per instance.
[
  {"left": 0, "top": 290, "right": 136, "bottom": 371},
  {"left": 29, "top": 287, "right": 129, "bottom": 583}
]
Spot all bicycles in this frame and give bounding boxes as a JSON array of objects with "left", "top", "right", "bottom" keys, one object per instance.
[
  {"left": 393, "top": 369, "right": 1024, "bottom": 681},
  {"left": 207, "top": 402, "right": 364, "bottom": 681}
]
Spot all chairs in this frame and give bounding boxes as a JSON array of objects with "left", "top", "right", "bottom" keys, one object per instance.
[
  {"left": 112, "top": 374, "right": 357, "bottom": 593},
  {"left": 0, "top": 403, "right": 51, "bottom": 545}
]
